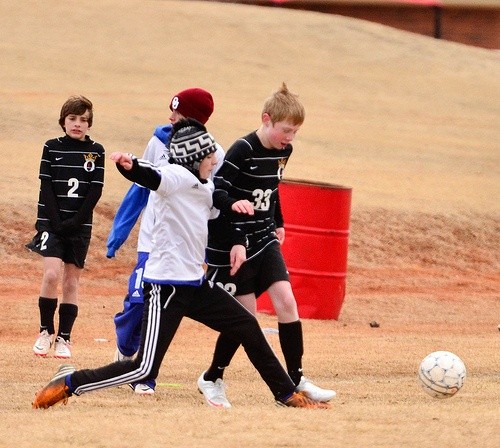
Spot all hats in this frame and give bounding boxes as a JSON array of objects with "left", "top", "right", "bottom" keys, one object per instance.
[
  {"left": 169, "top": 125, "right": 217, "bottom": 183},
  {"left": 169, "top": 87, "right": 214, "bottom": 123}
]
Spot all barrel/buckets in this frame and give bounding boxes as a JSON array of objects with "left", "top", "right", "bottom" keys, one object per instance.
[{"left": 256, "top": 176, "right": 353, "bottom": 321}]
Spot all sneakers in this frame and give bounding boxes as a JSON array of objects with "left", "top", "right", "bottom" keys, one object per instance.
[
  {"left": 54, "top": 336, "right": 71, "bottom": 358},
  {"left": 197, "top": 370, "right": 232, "bottom": 408},
  {"left": 33, "top": 329, "right": 55, "bottom": 356},
  {"left": 275, "top": 390, "right": 332, "bottom": 410},
  {"left": 296, "top": 376, "right": 336, "bottom": 403},
  {"left": 115, "top": 348, "right": 139, "bottom": 362},
  {"left": 134, "top": 384, "right": 154, "bottom": 394},
  {"left": 32, "top": 364, "right": 77, "bottom": 409}
]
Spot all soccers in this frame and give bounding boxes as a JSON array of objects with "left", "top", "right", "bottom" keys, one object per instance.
[{"left": 418, "top": 351, "right": 466, "bottom": 399}]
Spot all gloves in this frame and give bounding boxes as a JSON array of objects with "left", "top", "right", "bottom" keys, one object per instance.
[
  {"left": 55, "top": 217, "right": 77, "bottom": 232},
  {"left": 61, "top": 235, "right": 80, "bottom": 256}
]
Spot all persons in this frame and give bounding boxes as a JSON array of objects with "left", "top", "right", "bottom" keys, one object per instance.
[
  {"left": 197, "top": 82, "right": 335, "bottom": 408},
  {"left": 25, "top": 95, "right": 105, "bottom": 359},
  {"left": 106, "top": 88, "right": 226, "bottom": 394},
  {"left": 31, "top": 118, "right": 331, "bottom": 409}
]
